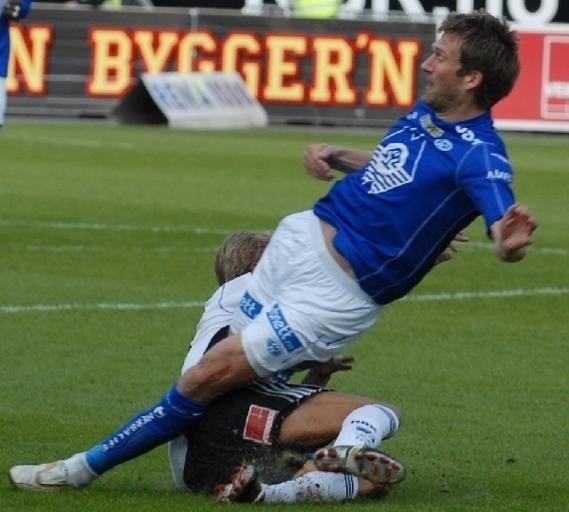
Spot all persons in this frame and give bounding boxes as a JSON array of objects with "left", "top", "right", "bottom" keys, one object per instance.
[
  {"left": 165, "top": 229, "right": 470, "bottom": 504},
  {"left": 6, "top": 7, "right": 539, "bottom": 492},
  {"left": 0, "top": 1, "right": 33, "bottom": 129}
]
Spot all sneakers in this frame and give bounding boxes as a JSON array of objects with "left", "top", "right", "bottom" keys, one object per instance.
[
  {"left": 312, "top": 441, "right": 405, "bottom": 484},
  {"left": 212, "top": 464, "right": 266, "bottom": 504},
  {"left": 7, "top": 458, "right": 87, "bottom": 491}
]
